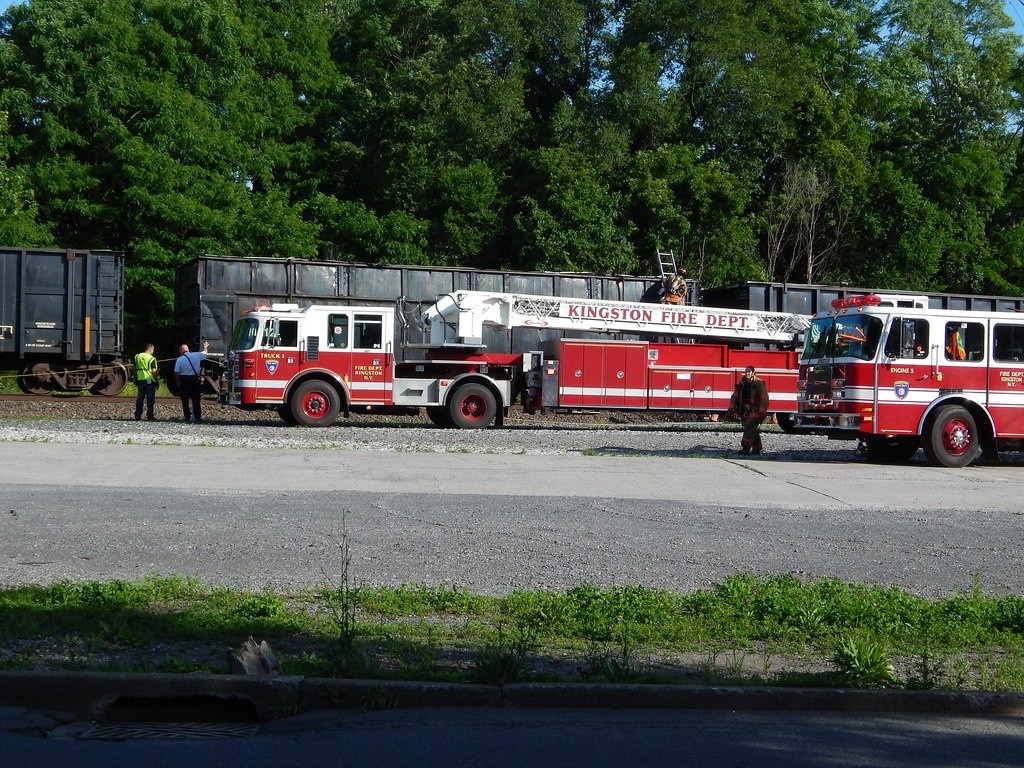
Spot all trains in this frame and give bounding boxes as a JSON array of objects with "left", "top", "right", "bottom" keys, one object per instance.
[{"left": 0, "top": 246, "right": 1024, "bottom": 397}]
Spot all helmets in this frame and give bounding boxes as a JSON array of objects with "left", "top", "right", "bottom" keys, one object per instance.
[{"left": 677, "top": 267, "right": 686, "bottom": 276}]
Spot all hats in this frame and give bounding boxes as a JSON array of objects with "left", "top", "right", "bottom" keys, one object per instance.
[{"left": 744, "top": 366, "right": 755, "bottom": 372}]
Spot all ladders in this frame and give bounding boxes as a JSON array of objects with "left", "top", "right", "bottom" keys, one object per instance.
[{"left": 655, "top": 244, "right": 679, "bottom": 282}]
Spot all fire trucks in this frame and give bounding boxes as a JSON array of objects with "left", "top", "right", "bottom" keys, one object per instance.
[
  {"left": 218, "top": 289, "right": 929, "bottom": 433},
  {"left": 794, "top": 295, "right": 1024, "bottom": 468}
]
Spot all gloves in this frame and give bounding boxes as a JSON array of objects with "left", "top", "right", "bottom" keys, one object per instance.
[{"left": 156, "top": 380, "right": 160, "bottom": 390}]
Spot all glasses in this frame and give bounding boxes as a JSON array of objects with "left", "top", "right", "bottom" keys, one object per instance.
[{"left": 837, "top": 337, "right": 844, "bottom": 343}]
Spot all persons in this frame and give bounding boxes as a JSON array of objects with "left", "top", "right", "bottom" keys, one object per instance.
[
  {"left": 134, "top": 343, "right": 159, "bottom": 422},
  {"left": 727, "top": 366, "right": 769, "bottom": 455},
  {"left": 664, "top": 266, "right": 687, "bottom": 305},
  {"left": 174, "top": 341, "right": 209, "bottom": 422}
]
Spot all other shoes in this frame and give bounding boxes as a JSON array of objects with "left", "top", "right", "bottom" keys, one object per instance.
[
  {"left": 194, "top": 418, "right": 203, "bottom": 422},
  {"left": 738, "top": 448, "right": 751, "bottom": 455},
  {"left": 751, "top": 444, "right": 763, "bottom": 454},
  {"left": 185, "top": 418, "right": 190, "bottom": 422},
  {"left": 135, "top": 417, "right": 142, "bottom": 421},
  {"left": 147, "top": 417, "right": 157, "bottom": 421}
]
